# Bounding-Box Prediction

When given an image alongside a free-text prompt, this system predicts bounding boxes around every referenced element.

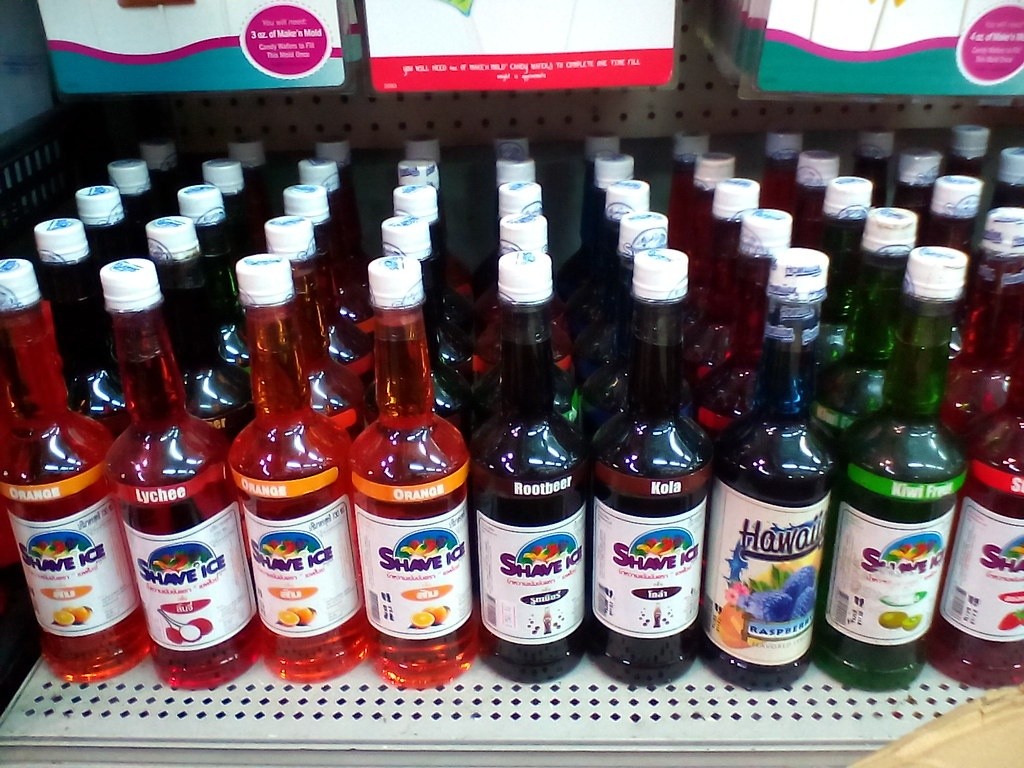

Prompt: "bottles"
[0,124,1024,690]
